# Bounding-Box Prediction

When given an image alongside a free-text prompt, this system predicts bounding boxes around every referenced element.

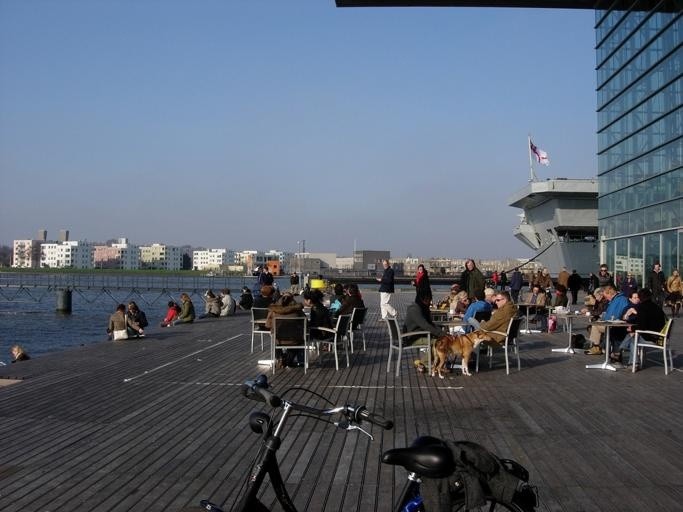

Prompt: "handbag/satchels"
[113,330,129,341]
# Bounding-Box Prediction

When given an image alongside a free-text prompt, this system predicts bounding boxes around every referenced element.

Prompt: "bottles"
[436,300,441,309]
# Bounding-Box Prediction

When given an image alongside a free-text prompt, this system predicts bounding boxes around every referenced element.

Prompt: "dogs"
[431,330,492,379]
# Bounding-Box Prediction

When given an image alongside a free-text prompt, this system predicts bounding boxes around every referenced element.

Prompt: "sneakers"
[583,346,601,355]
[392,312,398,319]
[378,318,385,322]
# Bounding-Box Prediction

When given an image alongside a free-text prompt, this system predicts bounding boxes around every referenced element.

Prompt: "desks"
[431,309,449,336]
[432,320,472,373]
[514,303,636,371]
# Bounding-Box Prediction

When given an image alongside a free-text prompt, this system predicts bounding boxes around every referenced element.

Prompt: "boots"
[672,306,675,317]
[676,304,681,318]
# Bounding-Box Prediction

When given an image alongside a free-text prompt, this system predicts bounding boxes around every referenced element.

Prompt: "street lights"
[296,240,302,288]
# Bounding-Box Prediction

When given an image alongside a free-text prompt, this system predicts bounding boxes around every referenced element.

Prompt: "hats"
[204,290,212,297]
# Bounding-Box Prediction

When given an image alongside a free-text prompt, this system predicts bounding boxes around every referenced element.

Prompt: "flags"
[530,139,548,166]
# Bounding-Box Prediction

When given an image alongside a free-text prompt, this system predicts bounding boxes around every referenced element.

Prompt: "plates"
[595,320,626,324]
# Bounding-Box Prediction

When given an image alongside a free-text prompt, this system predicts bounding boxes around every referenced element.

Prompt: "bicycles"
[200,374,539,512]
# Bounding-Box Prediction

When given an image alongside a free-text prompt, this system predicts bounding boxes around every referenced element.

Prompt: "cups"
[575,310,579,314]
[586,312,591,316]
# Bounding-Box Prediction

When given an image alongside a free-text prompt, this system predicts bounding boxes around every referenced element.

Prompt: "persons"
[450,259,519,349]
[160,293,195,328]
[111,302,148,340]
[253,285,365,367]
[403,259,444,346]
[290,272,299,293]
[510,266,582,316]
[198,286,254,318]
[377,260,399,322]
[10,344,30,363]
[586,262,683,368]
[258,266,274,286]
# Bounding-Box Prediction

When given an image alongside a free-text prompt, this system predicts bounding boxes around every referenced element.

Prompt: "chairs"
[250,307,369,374]
[383,317,433,377]
[474,316,523,375]
[631,317,675,376]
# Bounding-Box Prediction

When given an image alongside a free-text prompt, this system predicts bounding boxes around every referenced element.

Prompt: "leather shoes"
[609,351,622,361]
[536,327,544,332]
[528,319,536,324]
[623,362,639,369]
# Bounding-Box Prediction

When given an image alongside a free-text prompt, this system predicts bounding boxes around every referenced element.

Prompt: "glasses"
[600,270,606,272]
[496,299,504,302]
